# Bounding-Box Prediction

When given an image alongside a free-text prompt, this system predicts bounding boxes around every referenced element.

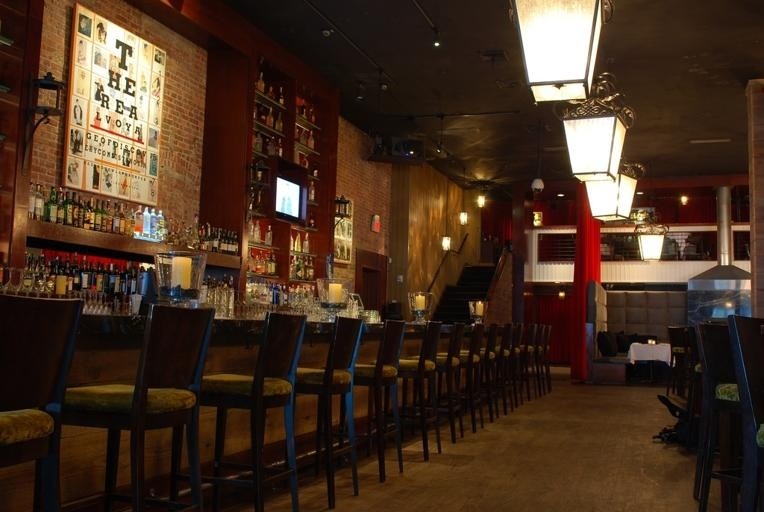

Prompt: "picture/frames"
[61,2,166,207]
[334,194,353,264]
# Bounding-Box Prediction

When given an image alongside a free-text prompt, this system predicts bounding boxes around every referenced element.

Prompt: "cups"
[11,268,24,295]
[24,272,35,297]
[122,296,132,316]
[317,277,352,321]
[408,291,433,325]
[114,296,122,316]
[80,291,112,315]
[35,276,46,298]
[0,268,12,295]
[469,300,488,326]
[132,295,143,314]
[46,278,56,298]
[154,249,206,307]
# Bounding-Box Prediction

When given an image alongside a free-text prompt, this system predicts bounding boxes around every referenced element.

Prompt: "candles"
[475,300,483,315]
[329,283,342,302]
[171,256,192,289]
[415,294,425,309]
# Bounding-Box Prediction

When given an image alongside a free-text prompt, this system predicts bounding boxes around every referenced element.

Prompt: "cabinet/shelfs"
[0,0,45,284]
[28,217,242,269]
[199,53,338,316]
[0,320,504,512]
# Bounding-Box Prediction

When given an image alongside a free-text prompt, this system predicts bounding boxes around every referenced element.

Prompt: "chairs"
[495,323,524,408]
[667,325,691,395]
[402,323,465,443]
[525,323,536,402]
[170,311,307,512]
[693,321,740,512]
[537,323,545,396]
[250,315,365,510]
[544,324,552,394]
[437,324,486,433]
[315,319,409,483]
[367,321,444,462]
[62,303,216,512]
[480,323,514,416]
[455,324,499,423]
[0,295,86,512]
[728,316,764,511]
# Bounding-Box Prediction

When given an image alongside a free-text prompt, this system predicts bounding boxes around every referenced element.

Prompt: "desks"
[627,343,676,368]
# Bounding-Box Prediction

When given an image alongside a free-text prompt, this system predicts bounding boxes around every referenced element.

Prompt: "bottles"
[256,72,265,93]
[48,187,59,223]
[249,186,264,212]
[297,101,317,124]
[124,210,131,234]
[266,107,273,128]
[63,192,73,226]
[277,138,283,158]
[134,205,143,237]
[74,256,82,290]
[291,235,294,251]
[273,284,280,305]
[309,217,315,228]
[113,203,120,233]
[0,253,9,291]
[66,258,73,293]
[28,182,34,219]
[201,272,235,287]
[72,192,78,227]
[280,285,284,304]
[282,285,289,303]
[78,196,83,228]
[254,221,261,243]
[308,181,314,202]
[199,225,239,256]
[119,203,125,235]
[248,248,267,275]
[56,192,64,223]
[290,256,315,280]
[130,208,136,236]
[248,281,272,303]
[249,220,254,241]
[107,200,113,233]
[143,207,150,238]
[303,233,309,252]
[84,200,91,229]
[271,252,278,276]
[156,210,165,239]
[140,266,145,271]
[279,87,284,105]
[251,160,265,181]
[286,285,315,305]
[256,133,276,157]
[275,111,282,131]
[148,267,154,272]
[265,254,272,276]
[265,225,272,247]
[101,200,107,232]
[151,208,157,238]
[25,255,64,275]
[90,198,95,230]
[82,260,137,294]
[294,233,302,251]
[35,184,45,220]
[267,87,274,98]
[308,130,314,150]
[95,199,101,231]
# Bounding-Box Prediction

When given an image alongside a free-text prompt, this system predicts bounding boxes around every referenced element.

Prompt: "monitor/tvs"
[275,176,308,223]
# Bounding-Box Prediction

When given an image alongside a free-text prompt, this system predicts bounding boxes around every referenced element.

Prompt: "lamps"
[584,155,646,222]
[629,211,669,262]
[477,190,486,208]
[440,236,451,253]
[458,212,467,226]
[508,0,614,106]
[553,55,635,182]
[532,178,545,201]
